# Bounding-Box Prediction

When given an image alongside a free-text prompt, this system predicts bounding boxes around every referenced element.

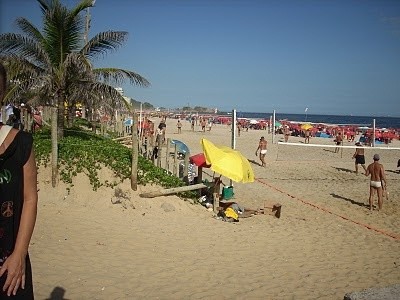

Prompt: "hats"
[373,153,379,159]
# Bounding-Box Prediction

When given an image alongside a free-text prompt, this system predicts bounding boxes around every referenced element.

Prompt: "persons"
[76,100,400,174]
[364,153,388,212]
[0,63,37,300]
[0,100,42,130]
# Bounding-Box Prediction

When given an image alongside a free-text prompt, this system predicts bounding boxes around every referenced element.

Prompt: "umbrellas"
[122,119,134,125]
[200,138,254,185]
[171,138,190,154]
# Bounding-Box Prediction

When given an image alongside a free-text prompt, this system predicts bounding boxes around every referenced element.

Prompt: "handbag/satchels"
[222,185,234,200]
